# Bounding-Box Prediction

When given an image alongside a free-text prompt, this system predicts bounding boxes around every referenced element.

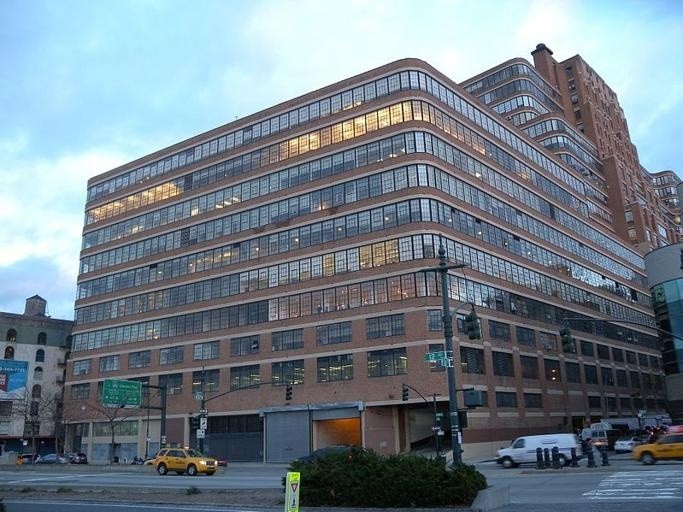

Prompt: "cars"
[142,446,217,476]
[15,452,86,465]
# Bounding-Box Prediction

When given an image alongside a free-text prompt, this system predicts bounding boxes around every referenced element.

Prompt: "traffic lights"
[284,383,293,401]
[466,311,481,340]
[401,386,408,403]
[558,328,573,353]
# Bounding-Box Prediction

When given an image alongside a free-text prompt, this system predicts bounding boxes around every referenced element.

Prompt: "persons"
[585,437,597,467]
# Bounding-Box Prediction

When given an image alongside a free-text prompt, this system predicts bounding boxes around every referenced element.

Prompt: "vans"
[581,413,683,463]
[495,432,584,470]
